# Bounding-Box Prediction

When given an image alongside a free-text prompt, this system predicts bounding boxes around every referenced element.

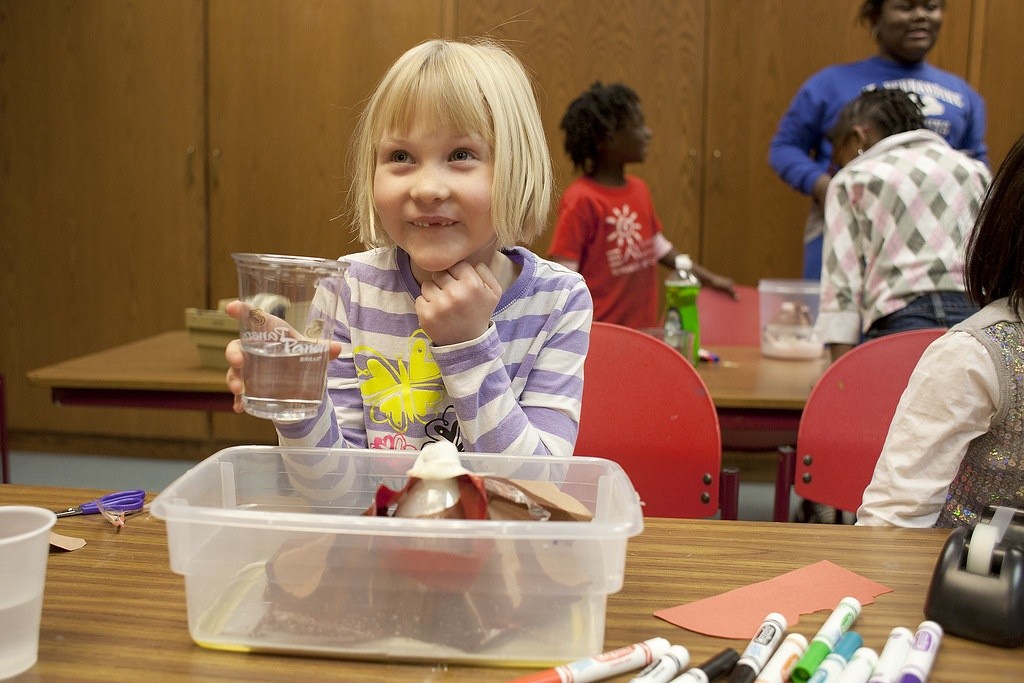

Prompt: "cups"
[758,279,825,360]
[232,253,350,421]
[0,506,59,680]
[645,328,695,365]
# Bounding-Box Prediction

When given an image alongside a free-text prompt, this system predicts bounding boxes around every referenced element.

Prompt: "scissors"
[53,489,145,517]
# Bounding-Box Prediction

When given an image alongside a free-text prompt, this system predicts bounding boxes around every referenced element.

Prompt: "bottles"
[665,254,699,368]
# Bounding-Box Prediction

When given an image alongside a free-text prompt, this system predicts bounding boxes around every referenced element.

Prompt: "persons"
[549,82,741,341]
[226,35,594,515]
[770,1,986,524]
[854,135,1024,529]
[820,89,994,366]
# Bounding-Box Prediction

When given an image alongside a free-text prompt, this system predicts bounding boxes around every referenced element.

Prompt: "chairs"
[793,325,950,515]
[698,286,808,348]
[573,322,723,520]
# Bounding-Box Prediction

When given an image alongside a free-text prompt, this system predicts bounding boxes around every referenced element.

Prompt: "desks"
[26,325,839,444]
[0,483,1024,683]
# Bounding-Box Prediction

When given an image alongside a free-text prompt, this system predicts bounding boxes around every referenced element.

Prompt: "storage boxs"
[148,443,646,665]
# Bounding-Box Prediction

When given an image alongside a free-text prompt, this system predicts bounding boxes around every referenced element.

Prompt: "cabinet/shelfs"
[0,0,444,457]
[442,0,977,317]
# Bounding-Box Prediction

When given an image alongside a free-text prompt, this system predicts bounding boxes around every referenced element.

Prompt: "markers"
[506,595,944,683]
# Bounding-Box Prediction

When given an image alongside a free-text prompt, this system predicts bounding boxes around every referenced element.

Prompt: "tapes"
[966,507,1017,576]
